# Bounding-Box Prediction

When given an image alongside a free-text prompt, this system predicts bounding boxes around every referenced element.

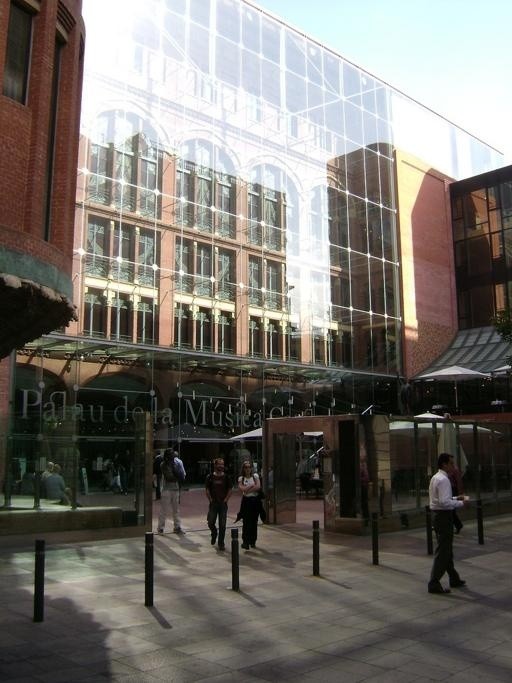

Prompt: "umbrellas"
[427,414,469,478]
[419,365,490,410]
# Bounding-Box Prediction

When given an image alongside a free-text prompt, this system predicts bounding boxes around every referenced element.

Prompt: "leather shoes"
[428,581,466,592]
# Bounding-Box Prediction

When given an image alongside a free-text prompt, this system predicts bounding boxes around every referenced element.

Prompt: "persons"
[103,449,133,495]
[152,449,187,536]
[427,452,466,594]
[205,457,234,551]
[234,461,266,550]
[19,461,73,506]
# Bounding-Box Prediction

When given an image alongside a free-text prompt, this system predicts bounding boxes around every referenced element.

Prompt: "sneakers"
[157,528,185,534]
[211,534,256,549]
